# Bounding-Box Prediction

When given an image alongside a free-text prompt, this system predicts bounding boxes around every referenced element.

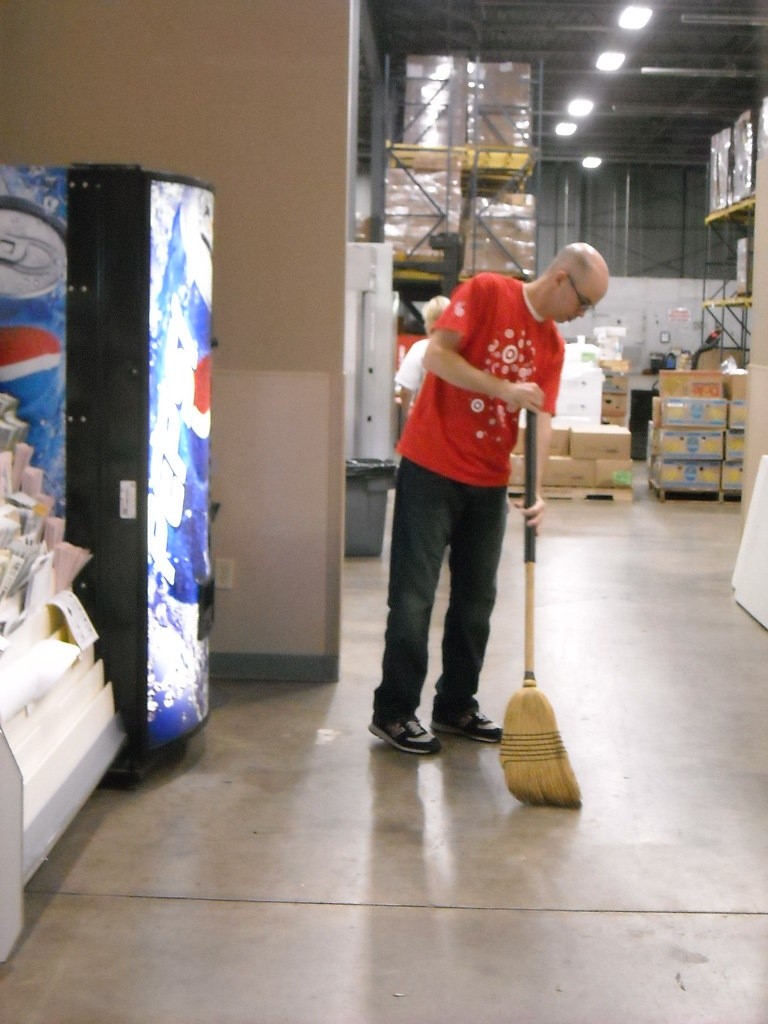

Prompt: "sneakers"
[369,713,442,755]
[430,700,503,743]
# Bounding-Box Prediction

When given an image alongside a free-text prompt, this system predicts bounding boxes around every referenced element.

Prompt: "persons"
[368,241,610,757]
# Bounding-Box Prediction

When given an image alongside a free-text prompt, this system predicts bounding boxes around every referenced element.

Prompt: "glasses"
[566,272,591,313]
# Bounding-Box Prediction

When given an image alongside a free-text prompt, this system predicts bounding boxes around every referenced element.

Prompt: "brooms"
[496,397,584,811]
